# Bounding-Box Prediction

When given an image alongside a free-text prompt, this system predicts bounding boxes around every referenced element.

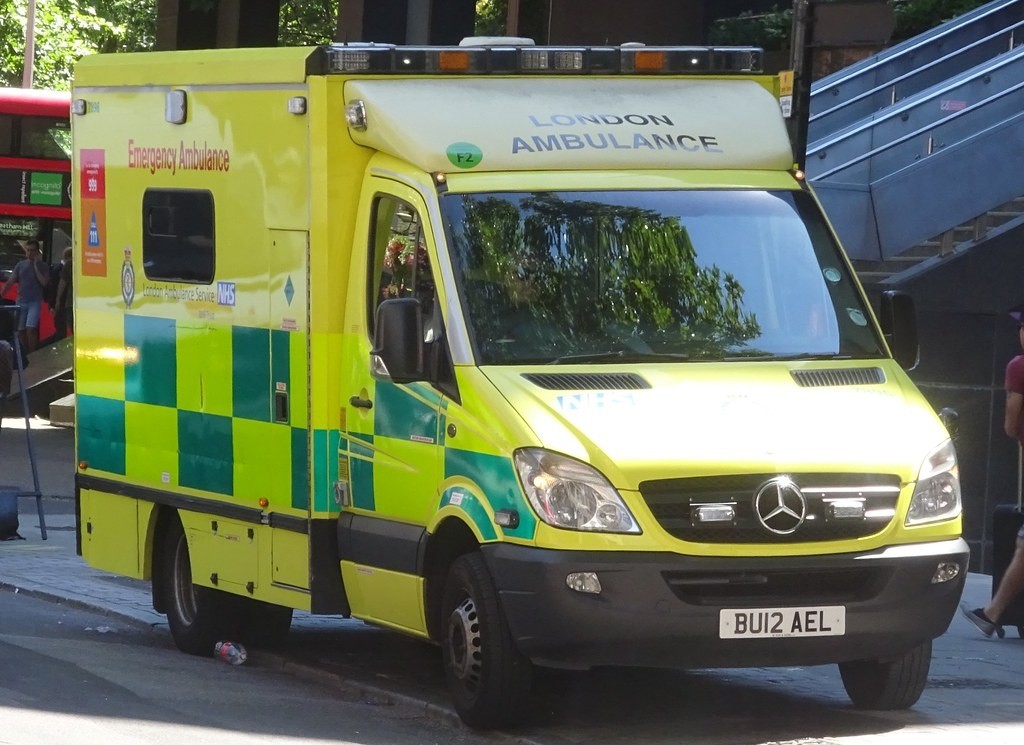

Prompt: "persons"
[503,244,552,311]
[49,248,73,337]
[958,313,1024,637]
[0,239,50,348]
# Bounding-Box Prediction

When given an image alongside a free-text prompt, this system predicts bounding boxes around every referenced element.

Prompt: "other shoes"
[957,598,996,638]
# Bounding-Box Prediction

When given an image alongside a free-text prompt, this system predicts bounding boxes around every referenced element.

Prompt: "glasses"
[1015,323,1024,333]
[508,267,545,283]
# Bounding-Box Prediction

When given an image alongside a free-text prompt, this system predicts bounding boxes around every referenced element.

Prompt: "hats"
[1009,303,1024,323]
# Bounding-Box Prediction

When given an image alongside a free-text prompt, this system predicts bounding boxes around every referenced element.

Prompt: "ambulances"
[70,36,971,734]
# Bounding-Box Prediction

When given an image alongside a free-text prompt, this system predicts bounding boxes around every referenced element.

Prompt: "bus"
[0,87,71,354]
